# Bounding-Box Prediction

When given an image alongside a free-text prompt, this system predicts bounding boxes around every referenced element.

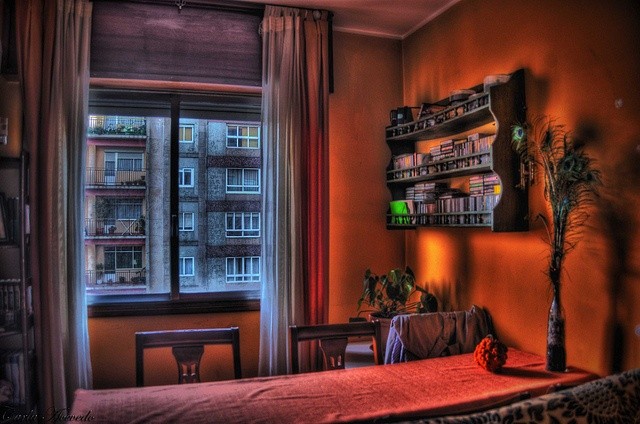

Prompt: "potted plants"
[96,263,104,284]
[91,195,113,236]
[120,174,146,188]
[135,215,145,235]
[355,264,439,361]
[507,110,606,373]
[108,225,116,234]
[136,268,146,284]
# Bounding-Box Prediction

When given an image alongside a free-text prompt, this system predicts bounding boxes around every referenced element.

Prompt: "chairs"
[133,326,242,387]
[285,318,384,376]
[384,304,494,365]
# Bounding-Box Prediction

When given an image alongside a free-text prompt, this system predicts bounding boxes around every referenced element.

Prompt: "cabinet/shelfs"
[0,152,42,423]
[385,67,530,232]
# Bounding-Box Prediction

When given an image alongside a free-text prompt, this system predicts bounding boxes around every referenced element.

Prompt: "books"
[389,173,502,224]
[389,134,497,178]
